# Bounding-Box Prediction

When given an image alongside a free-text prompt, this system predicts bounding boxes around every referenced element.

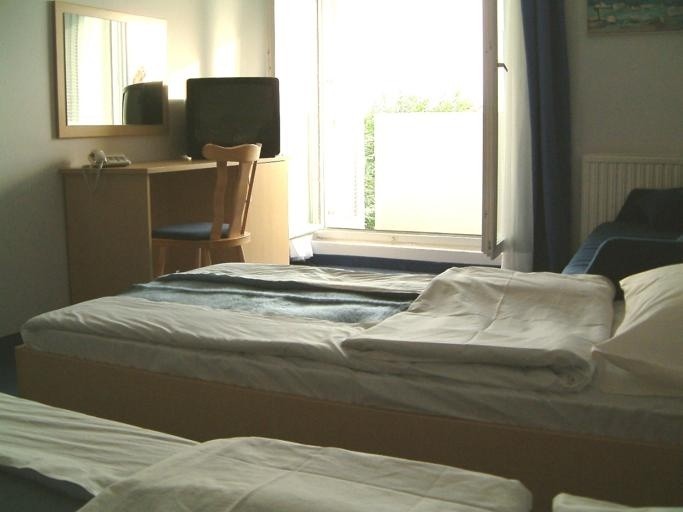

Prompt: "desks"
[59,153,290,308]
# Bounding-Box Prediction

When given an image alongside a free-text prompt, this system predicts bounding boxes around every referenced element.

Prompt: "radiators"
[573,151,683,250]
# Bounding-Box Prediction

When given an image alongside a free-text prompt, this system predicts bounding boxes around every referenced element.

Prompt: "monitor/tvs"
[122,80,162,123]
[185,77,280,157]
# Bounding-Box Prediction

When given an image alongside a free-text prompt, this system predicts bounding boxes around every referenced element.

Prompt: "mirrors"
[52,0,168,139]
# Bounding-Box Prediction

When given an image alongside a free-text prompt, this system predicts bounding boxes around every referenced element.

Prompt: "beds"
[11,257,683,511]
[0,385,680,511]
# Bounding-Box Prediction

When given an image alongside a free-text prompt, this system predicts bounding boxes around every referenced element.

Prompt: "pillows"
[588,261,683,389]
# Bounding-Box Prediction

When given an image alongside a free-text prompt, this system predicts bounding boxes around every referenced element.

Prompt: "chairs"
[147,139,262,281]
[562,185,683,297]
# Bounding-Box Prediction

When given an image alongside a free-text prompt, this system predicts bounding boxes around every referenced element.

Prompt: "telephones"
[88,149,131,168]
